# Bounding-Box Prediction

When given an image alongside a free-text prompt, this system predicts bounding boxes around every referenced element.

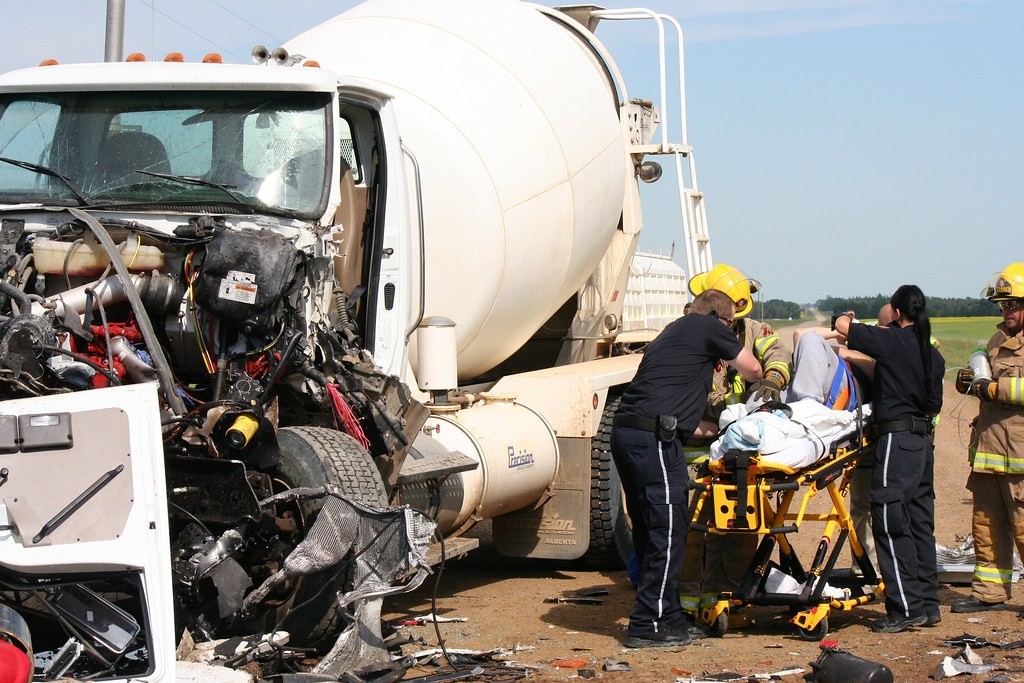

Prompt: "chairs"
[96,126,360,324]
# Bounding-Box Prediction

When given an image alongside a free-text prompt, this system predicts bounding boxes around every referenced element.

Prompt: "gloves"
[955,366,975,395]
[972,378,998,403]
[841,309,855,321]
[745,368,786,404]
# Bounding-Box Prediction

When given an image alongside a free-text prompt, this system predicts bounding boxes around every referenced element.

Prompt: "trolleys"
[672,430,897,640]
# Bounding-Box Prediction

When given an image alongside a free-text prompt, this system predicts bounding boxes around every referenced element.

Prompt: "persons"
[681,263,792,622]
[951,264,1024,618]
[610,290,763,648]
[834,285,945,632]
[711,304,938,580]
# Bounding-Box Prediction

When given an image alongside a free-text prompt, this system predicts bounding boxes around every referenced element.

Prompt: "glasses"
[995,300,1024,310]
[718,316,734,329]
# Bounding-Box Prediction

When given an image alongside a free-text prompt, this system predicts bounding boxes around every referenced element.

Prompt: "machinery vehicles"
[0,0,724,652]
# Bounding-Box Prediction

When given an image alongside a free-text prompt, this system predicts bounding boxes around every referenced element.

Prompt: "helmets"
[689,263,755,320]
[987,263,1024,303]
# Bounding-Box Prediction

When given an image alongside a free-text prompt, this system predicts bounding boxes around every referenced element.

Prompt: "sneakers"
[870,614,930,633]
[677,618,715,639]
[624,624,694,648]
[951,594,1007,613]
[918,609,942,627]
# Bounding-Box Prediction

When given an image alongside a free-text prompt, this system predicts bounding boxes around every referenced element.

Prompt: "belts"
[611,414,660,432]
[874,420,911,436]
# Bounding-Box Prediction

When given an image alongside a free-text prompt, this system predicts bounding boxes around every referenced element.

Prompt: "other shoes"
[847,564,864,584]
